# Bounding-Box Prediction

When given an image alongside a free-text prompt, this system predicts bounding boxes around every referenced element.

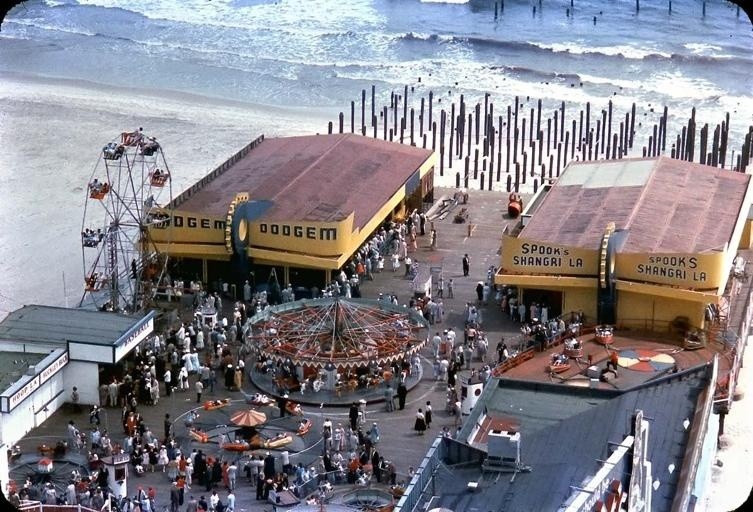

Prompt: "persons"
[82,228,94,247]
[8,386,434,512]
[103,302,110,311]
[334,213,437,292]
[131,259,136,276]
[89,178,107,192]
[153,169,164,180]
[489,264,584,367]
[463,253,470,276]
[100,275,488,429]
[91,274,97,287]
